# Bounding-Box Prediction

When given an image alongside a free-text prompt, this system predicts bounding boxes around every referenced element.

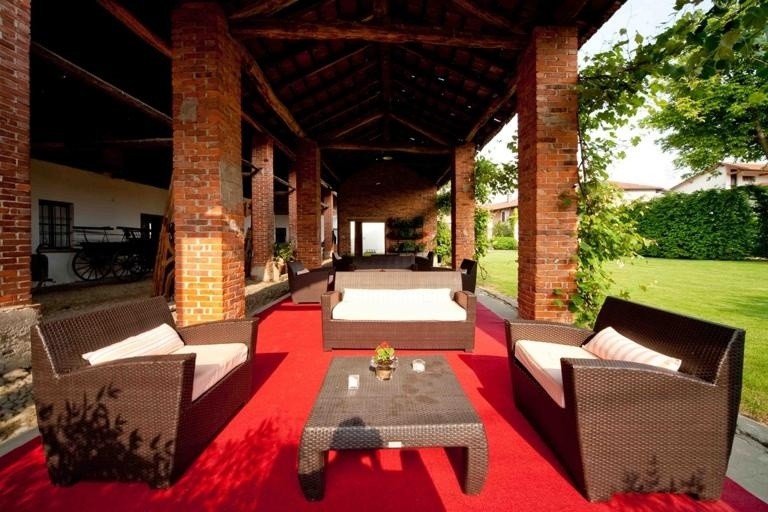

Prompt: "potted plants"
[274,239,297,274]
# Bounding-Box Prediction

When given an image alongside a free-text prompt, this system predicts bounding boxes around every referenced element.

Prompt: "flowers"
[374,341,395,365]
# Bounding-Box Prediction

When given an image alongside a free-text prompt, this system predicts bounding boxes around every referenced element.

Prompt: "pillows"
[80,322,186,366]
[581,326,682,372]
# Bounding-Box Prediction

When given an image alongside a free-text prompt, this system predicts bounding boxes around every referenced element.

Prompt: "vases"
[375,366,396,380]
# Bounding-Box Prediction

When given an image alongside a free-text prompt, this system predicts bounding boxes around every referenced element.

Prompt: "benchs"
[287,251,477,352]
[503,296,746,502]
[30,296,260,488]
